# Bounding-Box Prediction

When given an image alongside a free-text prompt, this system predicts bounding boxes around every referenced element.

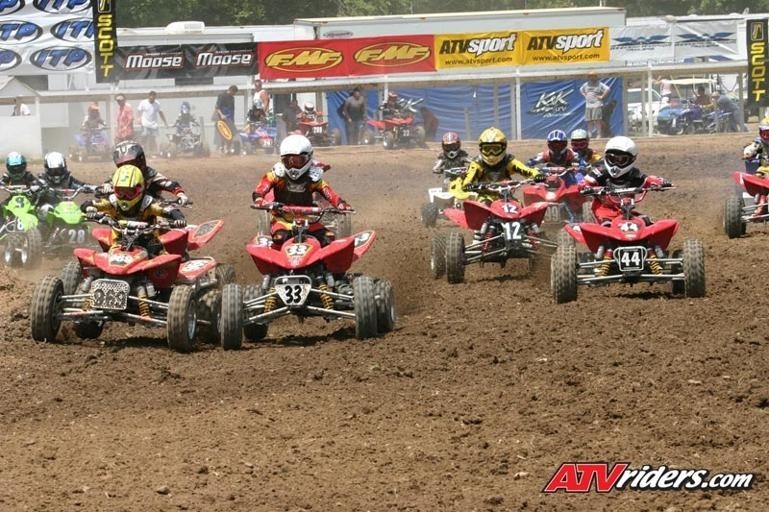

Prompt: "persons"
[12,96,31,117]
[82,78,421,153]
[430,129,674,229]
[250,136,350,247]
[418,105,440,143]
[1,150,41,220]
[97,140,190,206]
[712,90,746,131]
[743,118,769,177]
[654,74,673,105]
[81,167,188,256]
[696,85,710,105]
[33,151,96,206]
[579,71,612,138]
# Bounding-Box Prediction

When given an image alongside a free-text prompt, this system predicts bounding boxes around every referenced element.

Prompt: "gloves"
[534,174,546,183]
[463,183,475,192]
[659,181,672,191]
[102,182,113,195]
[176,192,192,209]
[255,196,269,209]
[173,219,186,228]
[584,186,596,196]
[337,202,352,212]
[86,205,98,220]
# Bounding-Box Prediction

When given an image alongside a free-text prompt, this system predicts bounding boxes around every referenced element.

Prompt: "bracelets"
[601,96,603,99]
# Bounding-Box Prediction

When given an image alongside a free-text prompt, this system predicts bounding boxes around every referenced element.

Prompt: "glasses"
[480,143,506,156]
[7,163,26,173]
[442,142,460,152]
[45,164,67,176]
[760,129,769,140]
[281,151,313,169]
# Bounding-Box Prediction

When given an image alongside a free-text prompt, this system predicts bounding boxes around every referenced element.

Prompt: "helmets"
[304,102,314,114]
[388,90,397,106]
[6,152,27,181]
[758,117,769,146]
[111,140,145,213]
[442,132,460,159]
[44,151,68,184]
[180,104,190,117]
[478,126,508,167]
[280,133,314,181]
[88,104,99,118]
[548,129,639,178]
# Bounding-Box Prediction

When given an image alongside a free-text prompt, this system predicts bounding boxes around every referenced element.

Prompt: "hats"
[585,71,600,79]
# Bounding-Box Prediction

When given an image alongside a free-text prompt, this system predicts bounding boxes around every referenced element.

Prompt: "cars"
[624,85,663,126]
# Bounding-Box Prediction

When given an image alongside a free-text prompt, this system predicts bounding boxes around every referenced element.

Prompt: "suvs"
[212,198,397,351]
[724,157,769,239]
[420,169,705,303]
[656,93,736,135]
[67,97,428,165]
[0,171,220,353]
